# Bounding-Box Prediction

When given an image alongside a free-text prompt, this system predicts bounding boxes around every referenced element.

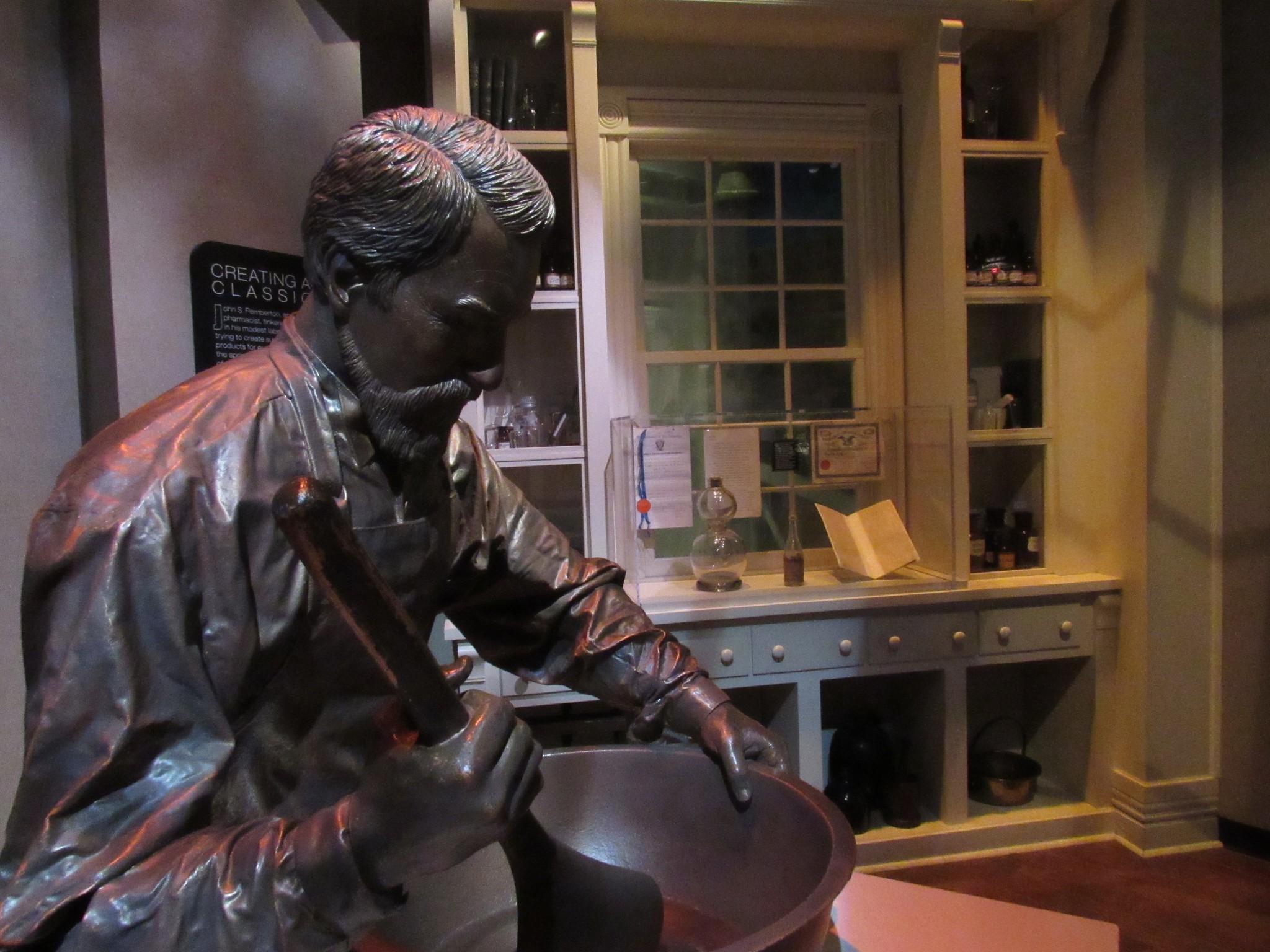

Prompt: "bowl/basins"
[343,741,854,952]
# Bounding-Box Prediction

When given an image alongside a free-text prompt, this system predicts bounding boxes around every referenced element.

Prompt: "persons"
[0,101,790,951]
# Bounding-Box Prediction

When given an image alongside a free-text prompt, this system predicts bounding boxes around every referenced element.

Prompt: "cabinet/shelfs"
[900,13,1062,581]
[408,569,1126,841]
[424,0,612,577]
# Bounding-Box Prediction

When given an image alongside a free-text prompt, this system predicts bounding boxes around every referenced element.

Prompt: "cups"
[885,772,921,826]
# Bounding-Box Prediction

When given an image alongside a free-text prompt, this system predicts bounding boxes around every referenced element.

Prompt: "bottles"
[781,516,805,587]
[970,507,1037,571]
[484,392,578,448]
[537,262,572,288]
[512,84,538,130]
[824,713,890,826]
[968,378,1032,431]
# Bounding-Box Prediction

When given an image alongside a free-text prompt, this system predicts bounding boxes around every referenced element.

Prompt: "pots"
[971,750,1042,804]
